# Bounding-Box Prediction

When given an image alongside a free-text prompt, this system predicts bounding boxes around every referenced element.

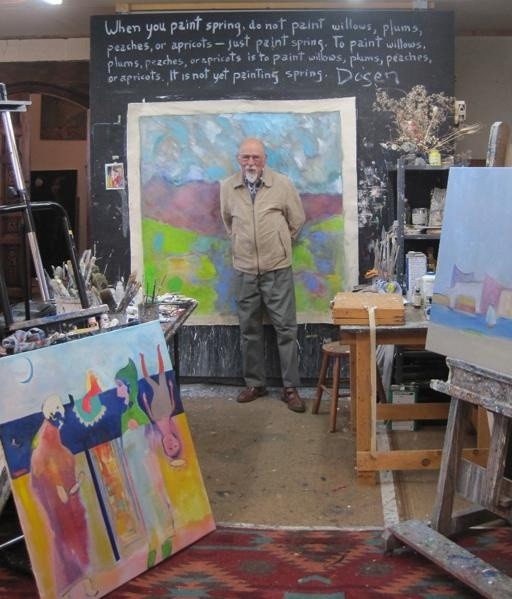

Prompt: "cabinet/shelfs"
[385,155,469,425]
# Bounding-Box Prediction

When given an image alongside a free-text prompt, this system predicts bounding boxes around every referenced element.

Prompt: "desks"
[340,295,493,485]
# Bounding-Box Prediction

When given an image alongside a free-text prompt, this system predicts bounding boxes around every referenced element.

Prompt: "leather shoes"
[281,386,305,413]
[236,385,267,403]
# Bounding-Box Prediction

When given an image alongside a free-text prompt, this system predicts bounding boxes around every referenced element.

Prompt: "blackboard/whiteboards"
[89,8,455,378]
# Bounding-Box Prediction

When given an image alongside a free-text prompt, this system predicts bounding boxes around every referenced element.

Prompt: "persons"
[219,137,308,413]
[116,356,178,568]
[139,345,182,460]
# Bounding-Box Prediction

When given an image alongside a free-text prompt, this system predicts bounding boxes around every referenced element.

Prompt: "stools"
[312,340,386,434]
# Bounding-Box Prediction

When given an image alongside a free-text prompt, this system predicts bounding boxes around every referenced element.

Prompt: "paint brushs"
[374,232,400,282]
[44,243,167,314]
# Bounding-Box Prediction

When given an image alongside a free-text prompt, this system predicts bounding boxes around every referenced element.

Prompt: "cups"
[412,208,428,225]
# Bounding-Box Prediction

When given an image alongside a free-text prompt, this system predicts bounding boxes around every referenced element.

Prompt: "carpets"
[0,522,512,599]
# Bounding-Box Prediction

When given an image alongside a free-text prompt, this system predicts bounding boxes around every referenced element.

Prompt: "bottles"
[403,198,411,225]
[107,281,124,304]
[428,149,442,166]
[87,310,109,331]
[411,289,422,308]
